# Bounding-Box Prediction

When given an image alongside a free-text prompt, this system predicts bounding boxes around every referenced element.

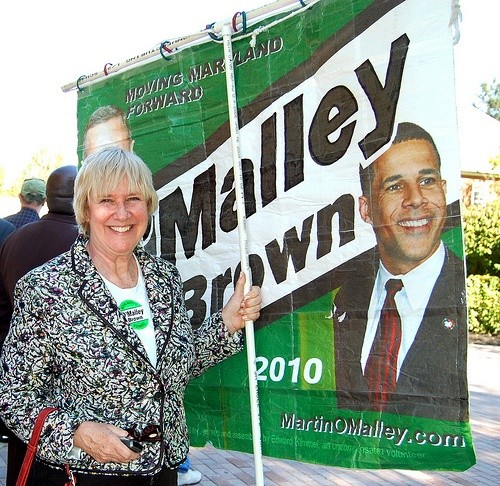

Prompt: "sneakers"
[177,470,201,486]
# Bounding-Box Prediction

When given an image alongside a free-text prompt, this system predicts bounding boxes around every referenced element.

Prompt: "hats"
[20,178,47,200]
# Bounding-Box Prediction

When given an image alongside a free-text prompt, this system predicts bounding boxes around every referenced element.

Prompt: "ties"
[363,278,404,416]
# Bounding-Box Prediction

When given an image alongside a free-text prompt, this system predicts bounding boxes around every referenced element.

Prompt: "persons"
[0,165,79,356]
[3,177,47,229]
[82,106,134,167]
[177,455,201,485]
[0,148,262,486]
[333,121,469,422]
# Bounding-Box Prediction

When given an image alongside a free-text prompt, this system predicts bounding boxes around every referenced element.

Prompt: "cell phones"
[120,439,143,453]
